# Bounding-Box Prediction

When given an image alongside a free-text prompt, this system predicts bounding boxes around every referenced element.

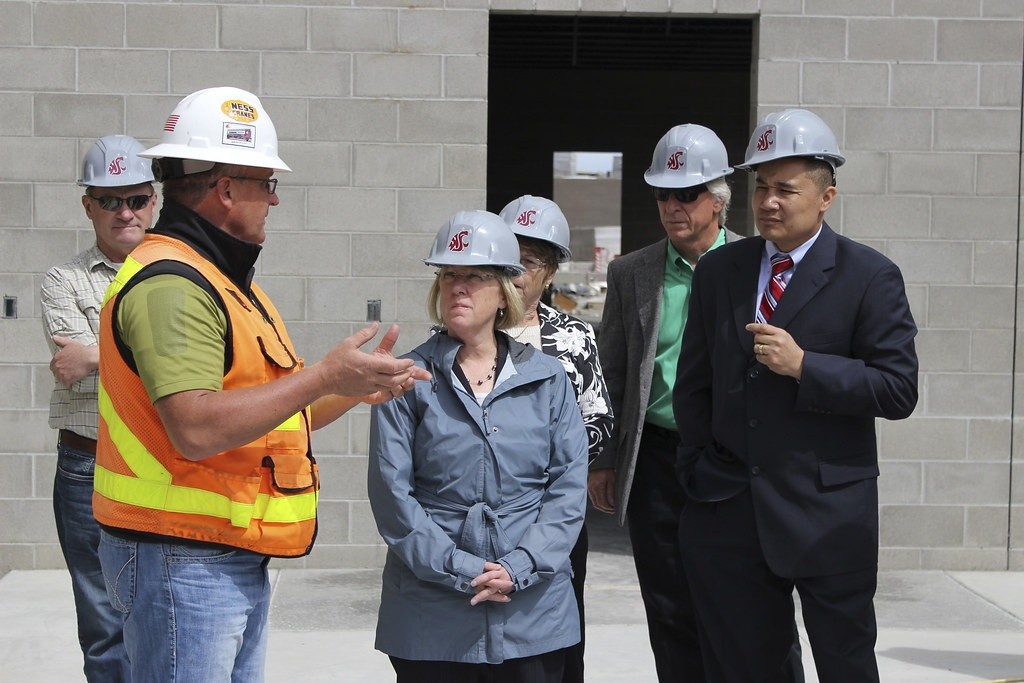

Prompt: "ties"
[755,253,794,325]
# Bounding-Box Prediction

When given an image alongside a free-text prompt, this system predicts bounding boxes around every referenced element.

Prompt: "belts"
[60,429,97,455]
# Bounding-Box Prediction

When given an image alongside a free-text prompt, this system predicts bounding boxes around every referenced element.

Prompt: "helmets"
[643,124,734,189]
[137,87,293,183]
[77,135,155,187]
[734,108,846,170]
[499,195,572,263]
[421,210,526,277]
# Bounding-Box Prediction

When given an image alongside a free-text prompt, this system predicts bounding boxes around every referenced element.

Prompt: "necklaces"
[465,355,499,386]
[503,324,530,339]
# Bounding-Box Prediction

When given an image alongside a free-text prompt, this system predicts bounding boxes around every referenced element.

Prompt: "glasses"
[434,268,495,287]
[209,175,278,195]
[653,186,708,203]
[520,254,546,270]
[87,193,152,211]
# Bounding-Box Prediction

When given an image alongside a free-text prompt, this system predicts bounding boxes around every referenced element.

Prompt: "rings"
[758,343,764,355]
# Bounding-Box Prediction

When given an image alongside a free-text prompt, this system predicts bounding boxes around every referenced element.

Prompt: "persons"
[91,87,432,683]
[671,108,919,683]
[40,134,159,683]
[368,209,590,683]
[497,195,614,683]
[597,123,749,683]
